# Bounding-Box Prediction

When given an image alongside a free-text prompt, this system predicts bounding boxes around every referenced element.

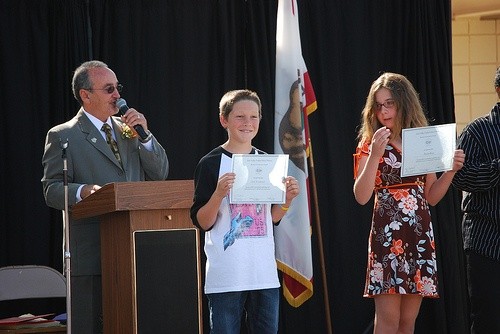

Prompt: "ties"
[99,123,122,165]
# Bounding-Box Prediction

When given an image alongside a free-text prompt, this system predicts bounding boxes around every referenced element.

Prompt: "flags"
[273,0,317,308]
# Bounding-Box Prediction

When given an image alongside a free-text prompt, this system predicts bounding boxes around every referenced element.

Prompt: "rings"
[137,114,140,119]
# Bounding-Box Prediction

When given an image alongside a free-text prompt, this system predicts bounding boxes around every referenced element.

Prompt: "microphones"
[115,98,149,140]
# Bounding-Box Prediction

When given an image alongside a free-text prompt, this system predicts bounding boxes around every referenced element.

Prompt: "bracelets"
[279,204,289,211]
[138,130,152,143]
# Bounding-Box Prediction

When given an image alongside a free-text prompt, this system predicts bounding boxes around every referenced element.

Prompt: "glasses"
[84,84,123,93]
[373,101,395,111]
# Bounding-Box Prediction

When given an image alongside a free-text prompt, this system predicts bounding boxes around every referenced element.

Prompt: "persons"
[450,66,500,334]
[191,89,300,334]
[42,61,169,334]
[353,73,465,334]
